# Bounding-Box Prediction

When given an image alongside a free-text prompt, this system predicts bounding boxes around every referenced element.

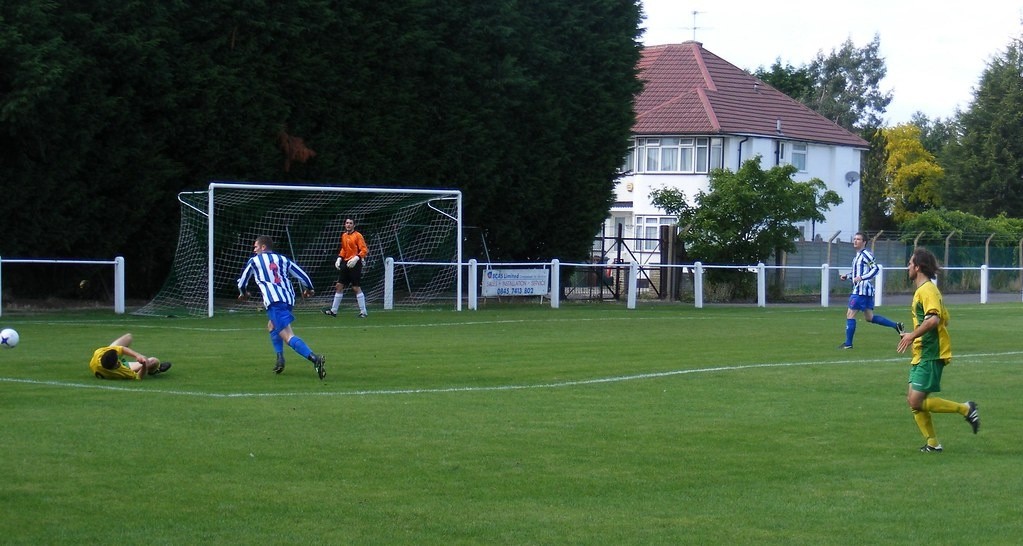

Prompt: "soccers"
[0,328,20,349]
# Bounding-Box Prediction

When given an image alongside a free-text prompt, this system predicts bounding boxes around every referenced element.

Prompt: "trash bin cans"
[570,256,611,287]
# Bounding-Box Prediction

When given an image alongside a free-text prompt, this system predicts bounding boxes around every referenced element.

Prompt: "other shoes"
[148,362,171,375]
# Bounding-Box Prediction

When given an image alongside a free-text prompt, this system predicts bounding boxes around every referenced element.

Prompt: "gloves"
[335,257,342,270]
[346,256,360,268]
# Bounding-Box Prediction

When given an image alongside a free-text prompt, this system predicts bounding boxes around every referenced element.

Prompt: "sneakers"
[895,322,905,339]
[315,355,326,379]
[964,400,978,434]
[835,343,853,349]
[273,363,284,374]
[320,307,336,318]
[359,313,367,319]
[919,444,942,453]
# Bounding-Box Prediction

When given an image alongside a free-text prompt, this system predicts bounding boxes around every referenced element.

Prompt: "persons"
[237,235,327,380]
[896,246,981,455]
[836,232,905,350]
[89,332,171,380]
[322,217,369,318]
